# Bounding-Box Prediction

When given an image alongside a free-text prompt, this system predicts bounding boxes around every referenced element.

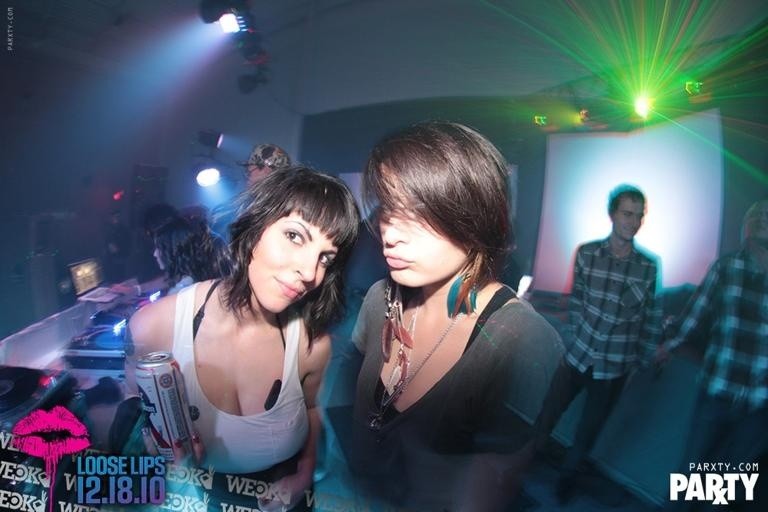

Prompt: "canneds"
[134,351,199,462]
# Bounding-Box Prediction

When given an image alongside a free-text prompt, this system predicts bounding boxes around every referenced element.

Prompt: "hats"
[235,144,291,171]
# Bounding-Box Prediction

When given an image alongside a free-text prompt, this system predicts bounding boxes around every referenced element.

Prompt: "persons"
[103,118,768,512]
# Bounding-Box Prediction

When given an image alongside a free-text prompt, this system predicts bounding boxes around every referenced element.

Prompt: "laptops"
[68,257,124,303]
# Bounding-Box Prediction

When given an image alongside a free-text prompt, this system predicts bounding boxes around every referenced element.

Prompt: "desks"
[1,278,137,414]
